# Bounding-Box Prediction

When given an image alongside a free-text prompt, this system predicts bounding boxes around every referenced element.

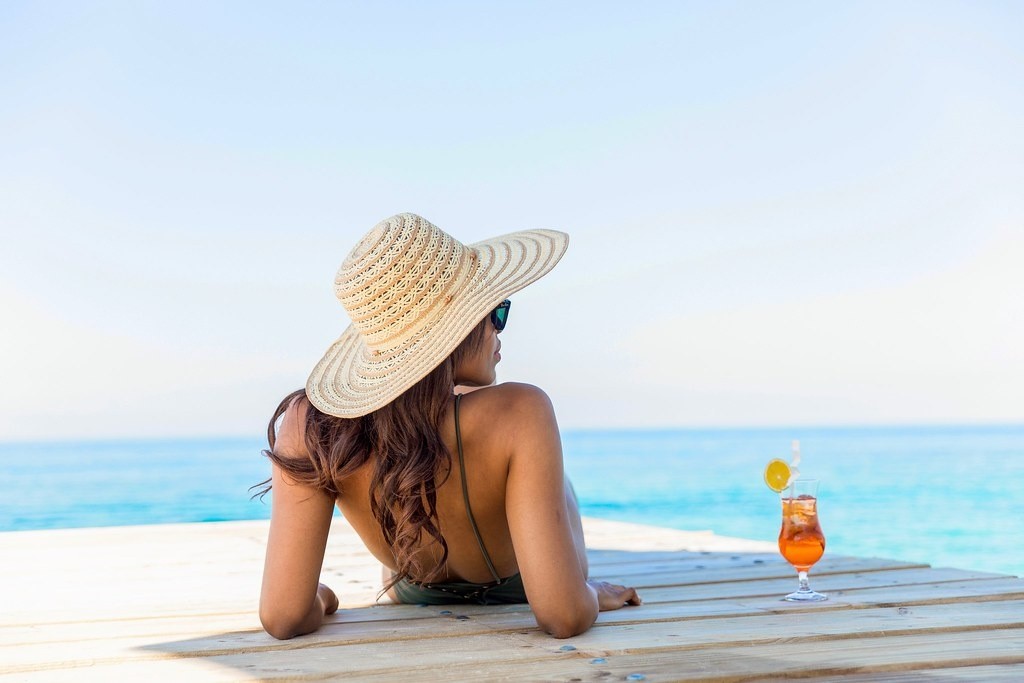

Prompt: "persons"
[247,212,642,640]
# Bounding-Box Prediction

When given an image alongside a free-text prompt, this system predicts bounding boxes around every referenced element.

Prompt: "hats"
[305,213,569,419]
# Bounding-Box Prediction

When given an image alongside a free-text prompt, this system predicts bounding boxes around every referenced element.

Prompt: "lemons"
[764,459,791,492]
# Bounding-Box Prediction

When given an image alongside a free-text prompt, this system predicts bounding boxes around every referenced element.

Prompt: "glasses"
[490,300,512,331]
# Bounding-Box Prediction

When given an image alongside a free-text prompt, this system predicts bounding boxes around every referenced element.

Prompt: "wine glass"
[779,477,829,603]
